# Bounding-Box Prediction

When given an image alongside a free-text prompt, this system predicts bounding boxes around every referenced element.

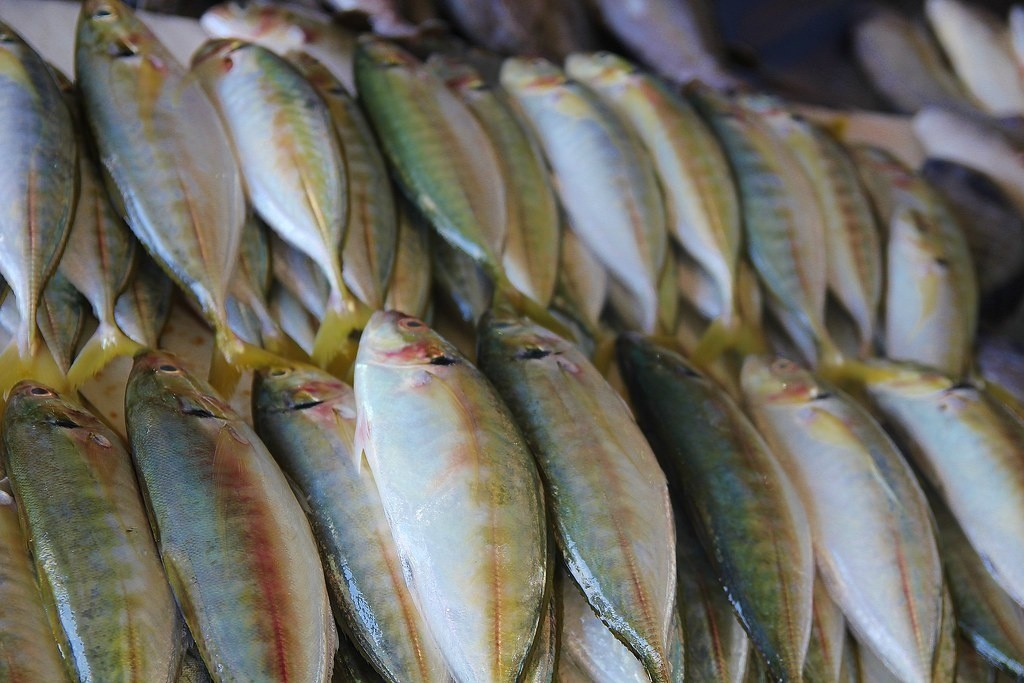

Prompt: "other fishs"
[0,0,1022,683]
